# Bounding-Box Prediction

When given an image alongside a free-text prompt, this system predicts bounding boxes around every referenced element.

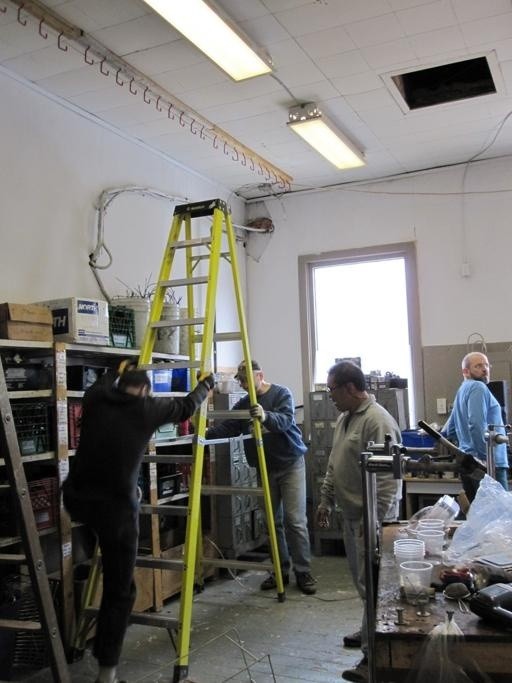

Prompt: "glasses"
[326,385,344,393]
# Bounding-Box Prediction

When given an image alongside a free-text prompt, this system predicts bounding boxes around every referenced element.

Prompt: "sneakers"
[342,659,369,683]
[343,631,361,647]
[261,572,289,590]
[296,573,318,594]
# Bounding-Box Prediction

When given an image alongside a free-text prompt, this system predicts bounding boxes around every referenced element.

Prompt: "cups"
[390,516,446,607]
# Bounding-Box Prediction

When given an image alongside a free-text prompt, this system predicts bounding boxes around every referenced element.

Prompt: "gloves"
[249,403,266,423]
[197,371,217,392]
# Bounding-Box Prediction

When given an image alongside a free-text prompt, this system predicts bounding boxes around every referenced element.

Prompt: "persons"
[319,362,406,681]
[439,350,510,502]
[204,358,313,594]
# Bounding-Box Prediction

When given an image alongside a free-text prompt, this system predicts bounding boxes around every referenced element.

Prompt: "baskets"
[0,572,62,681]
[10,402,50,456]
[137,463,150,499]
[69,402,83,449]
[0,477,60,537]
[109,305,136,348]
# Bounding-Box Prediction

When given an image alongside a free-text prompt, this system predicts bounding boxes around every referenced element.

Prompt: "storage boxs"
[37,298,114,347]
[5,300,56,344]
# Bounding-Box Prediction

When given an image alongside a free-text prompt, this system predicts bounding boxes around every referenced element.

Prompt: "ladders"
[69,199,284,682]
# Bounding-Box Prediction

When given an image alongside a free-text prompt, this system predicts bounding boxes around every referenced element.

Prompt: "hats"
[234,360,261,378]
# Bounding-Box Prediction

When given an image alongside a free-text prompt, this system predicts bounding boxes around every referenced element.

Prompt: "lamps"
[289,101,367,170]
[147,0,273,87]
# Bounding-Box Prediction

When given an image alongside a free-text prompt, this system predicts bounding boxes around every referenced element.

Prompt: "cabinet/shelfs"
[2,341,69,683]
[307,384,404,554]
[203,431,269,573]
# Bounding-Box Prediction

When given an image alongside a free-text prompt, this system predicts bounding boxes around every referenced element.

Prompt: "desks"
[403,476,465,520]
[369,519,512,682]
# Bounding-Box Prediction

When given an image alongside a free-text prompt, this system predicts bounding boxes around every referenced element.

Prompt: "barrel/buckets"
[400,428,449,460]
[110,298,151,350]
[179,306,203,357]
[151,302,180,356]
